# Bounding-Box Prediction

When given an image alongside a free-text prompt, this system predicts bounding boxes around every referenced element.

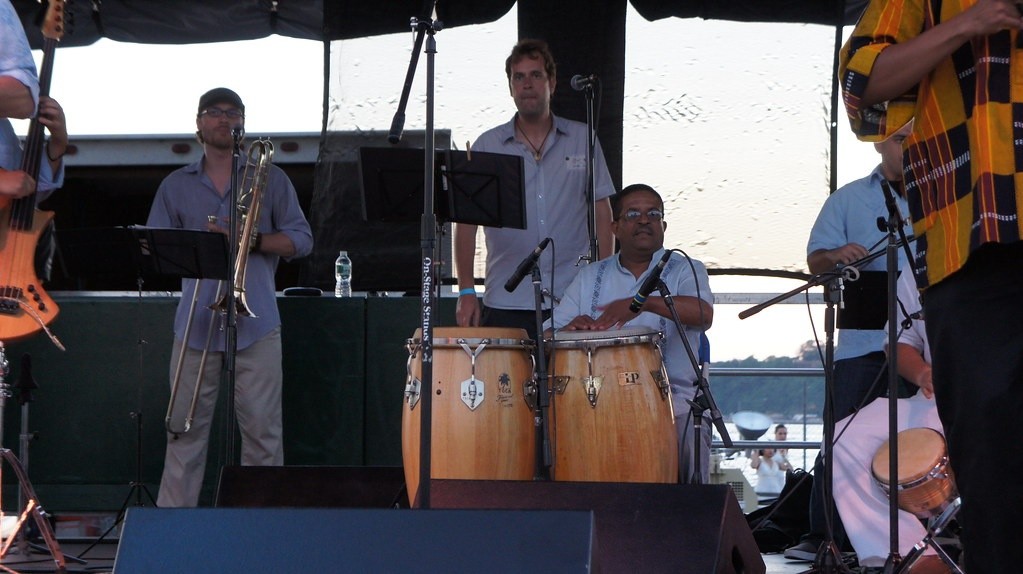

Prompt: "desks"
[3,291,486,515]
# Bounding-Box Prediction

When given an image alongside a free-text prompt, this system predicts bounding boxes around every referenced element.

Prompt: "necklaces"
[516,120,552,160]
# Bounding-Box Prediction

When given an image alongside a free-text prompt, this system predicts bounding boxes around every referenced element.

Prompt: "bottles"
[335,250,351,297]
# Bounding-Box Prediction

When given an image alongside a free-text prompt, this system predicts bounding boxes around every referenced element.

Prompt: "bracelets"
[254,230,263,256]
[45,139,67,162]
[458,287,476,296]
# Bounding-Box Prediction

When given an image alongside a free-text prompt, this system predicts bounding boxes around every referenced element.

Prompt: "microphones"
[910,309,926,319]
[629,250,672,313]
[231,125,243,139]
[503,237,549,293]
[571,74,598,91]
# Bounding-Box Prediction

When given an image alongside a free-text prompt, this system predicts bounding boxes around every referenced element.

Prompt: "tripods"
[73,412,157,557]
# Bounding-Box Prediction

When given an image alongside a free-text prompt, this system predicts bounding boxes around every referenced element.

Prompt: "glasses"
[198,106,245,120]
[612,208,665,223]
[776,432,786,436]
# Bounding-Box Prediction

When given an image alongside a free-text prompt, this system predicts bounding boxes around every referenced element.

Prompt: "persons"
[810,0,1023,574]
[542,184,712,484]
[806,115,956,574]
[146,86,313,508]
[752,440,785,493]
[455,39,619,340]
[0,0,68,552]
[770,423,793,473]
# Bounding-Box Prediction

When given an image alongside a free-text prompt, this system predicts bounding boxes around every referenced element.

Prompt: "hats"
[198,87,243,114]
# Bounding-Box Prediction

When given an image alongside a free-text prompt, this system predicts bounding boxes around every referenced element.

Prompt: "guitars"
[1,1,78,351]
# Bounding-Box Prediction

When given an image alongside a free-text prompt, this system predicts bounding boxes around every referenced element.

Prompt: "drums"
[546,325,680,485]
[400,325,540,509]
[870,426,959,520]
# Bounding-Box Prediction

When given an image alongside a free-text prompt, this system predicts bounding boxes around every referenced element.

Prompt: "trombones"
[162,137,277,435]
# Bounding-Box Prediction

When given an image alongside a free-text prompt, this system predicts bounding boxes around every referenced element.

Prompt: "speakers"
[114,463,766,574]
